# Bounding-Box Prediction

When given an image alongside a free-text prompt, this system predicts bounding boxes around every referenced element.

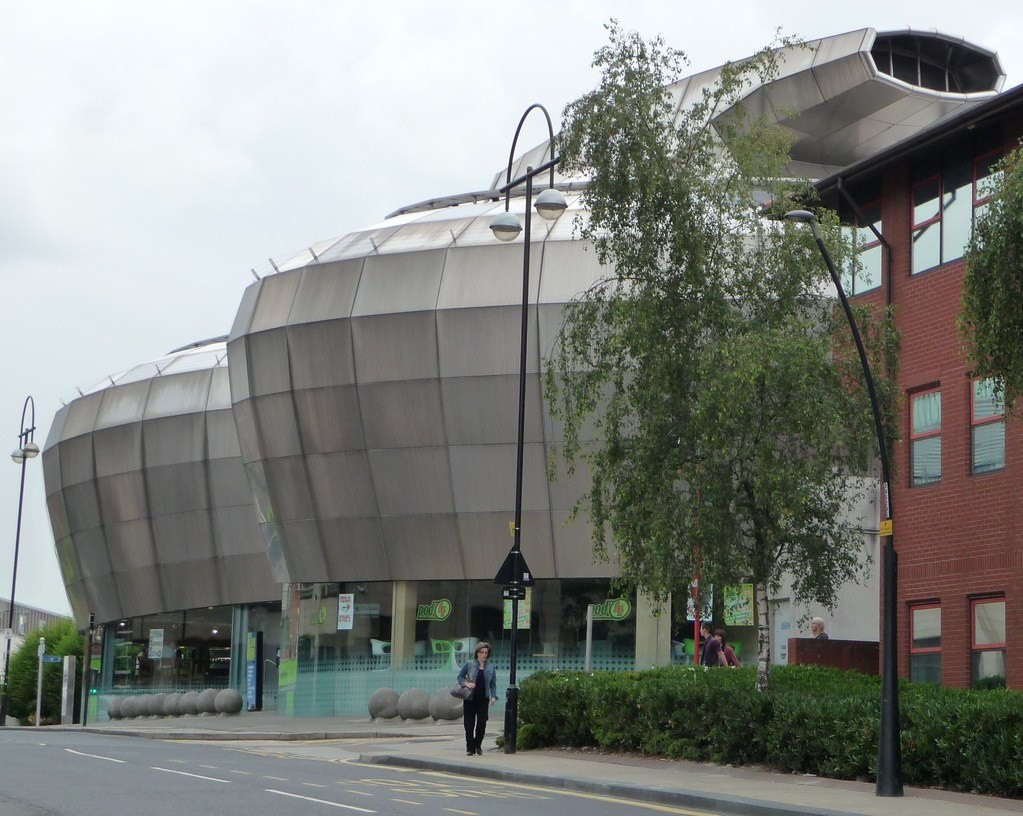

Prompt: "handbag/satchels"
[450,660,474,701]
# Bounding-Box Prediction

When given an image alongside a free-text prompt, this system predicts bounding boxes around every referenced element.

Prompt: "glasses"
[479,651,489,654]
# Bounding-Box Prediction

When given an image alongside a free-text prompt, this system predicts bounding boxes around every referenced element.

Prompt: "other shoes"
[476,745,482,755]
[467,751,473,756]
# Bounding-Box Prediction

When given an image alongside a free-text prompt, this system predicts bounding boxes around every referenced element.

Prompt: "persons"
[457,642,498,755]
[714,630,740,666]
[699,621,727,666]
[810,617,828,639]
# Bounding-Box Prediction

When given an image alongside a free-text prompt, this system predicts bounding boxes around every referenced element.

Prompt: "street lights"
[0,395,41,729]
[789,209,904,798]
[488,103,571,750]
